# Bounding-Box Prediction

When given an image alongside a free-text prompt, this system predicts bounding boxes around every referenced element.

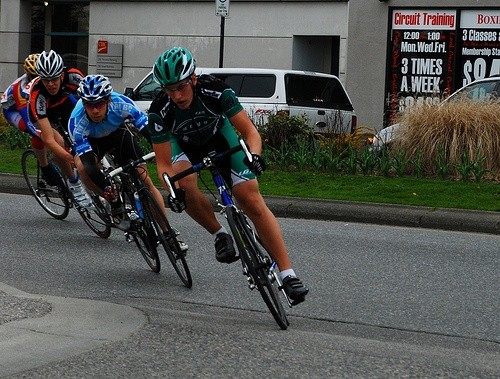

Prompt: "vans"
[123,67,356,142]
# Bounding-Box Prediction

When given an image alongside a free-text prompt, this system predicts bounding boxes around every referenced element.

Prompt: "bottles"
[134,191,144,219]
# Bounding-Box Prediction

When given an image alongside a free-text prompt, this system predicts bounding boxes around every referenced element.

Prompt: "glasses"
[42,77,60,83]
[83,100,109,109]
[161,78,190,92]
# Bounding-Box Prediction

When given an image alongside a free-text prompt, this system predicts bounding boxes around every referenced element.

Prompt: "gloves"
[243,154,267,177]
[168,187,187,213]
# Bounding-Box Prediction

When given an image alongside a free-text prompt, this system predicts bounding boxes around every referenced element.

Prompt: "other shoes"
[39,162,60,186]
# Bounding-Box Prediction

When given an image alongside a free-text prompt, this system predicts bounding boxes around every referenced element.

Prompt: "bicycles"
[52,117,126,238]
[162,134,305,330]
[102,151,193,288]
[21,149,73,219]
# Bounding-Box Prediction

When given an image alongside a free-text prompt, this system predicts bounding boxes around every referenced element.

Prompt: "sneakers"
[165,233,188,253]
[214,232,236,262]
[67,178,92,207]
[110,200,131,231]
[283,274,309,299]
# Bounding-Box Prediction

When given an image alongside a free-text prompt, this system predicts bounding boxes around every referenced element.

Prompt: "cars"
[366,77,500,152]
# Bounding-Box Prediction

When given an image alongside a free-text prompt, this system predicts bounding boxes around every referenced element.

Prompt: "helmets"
[77,74,113,104]
[152,46,196,87]
[35,50,65,80]
[23,54,43,76]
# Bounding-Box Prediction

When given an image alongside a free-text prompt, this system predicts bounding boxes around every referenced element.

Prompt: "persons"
[148,47,309,299]
[31,50,92,206]
[68,75,189,253]
[0,53,63,186]
